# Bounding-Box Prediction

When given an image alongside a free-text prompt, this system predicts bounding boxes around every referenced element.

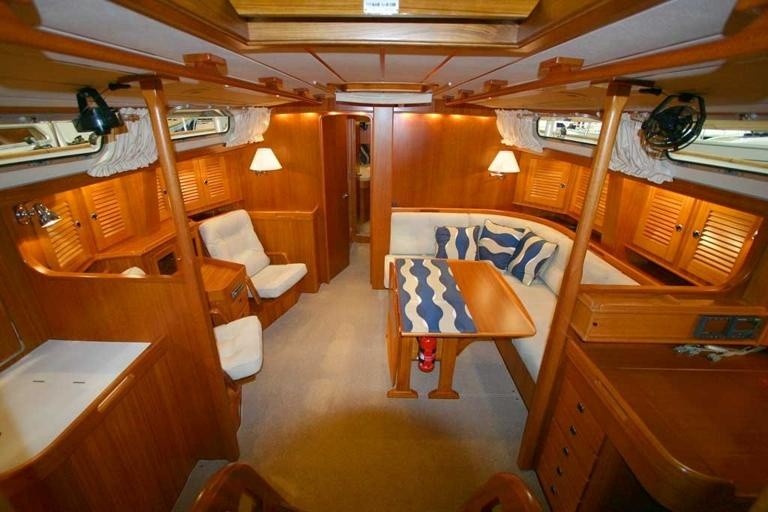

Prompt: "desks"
[386,257,537,399]
[534,339,768,511]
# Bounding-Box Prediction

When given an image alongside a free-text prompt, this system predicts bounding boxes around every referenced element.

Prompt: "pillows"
[506,225,558,286]
[478,218,523,272]
[435,226,480,261]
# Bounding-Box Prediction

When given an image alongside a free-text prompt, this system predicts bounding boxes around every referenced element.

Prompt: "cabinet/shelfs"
[198,256,250,326]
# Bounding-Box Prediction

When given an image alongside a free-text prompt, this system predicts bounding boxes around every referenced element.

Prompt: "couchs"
[389,205,667,413]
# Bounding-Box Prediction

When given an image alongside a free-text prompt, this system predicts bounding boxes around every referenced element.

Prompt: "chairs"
[101,267,264,383]
[195,209,308,330]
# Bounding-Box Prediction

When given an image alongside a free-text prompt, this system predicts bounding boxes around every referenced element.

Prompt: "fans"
[638,93,707,158]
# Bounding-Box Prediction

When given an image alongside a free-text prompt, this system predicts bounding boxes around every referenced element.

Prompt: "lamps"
[249,147,282,176]
[15,203,62,228]
[487,150,521,178]
